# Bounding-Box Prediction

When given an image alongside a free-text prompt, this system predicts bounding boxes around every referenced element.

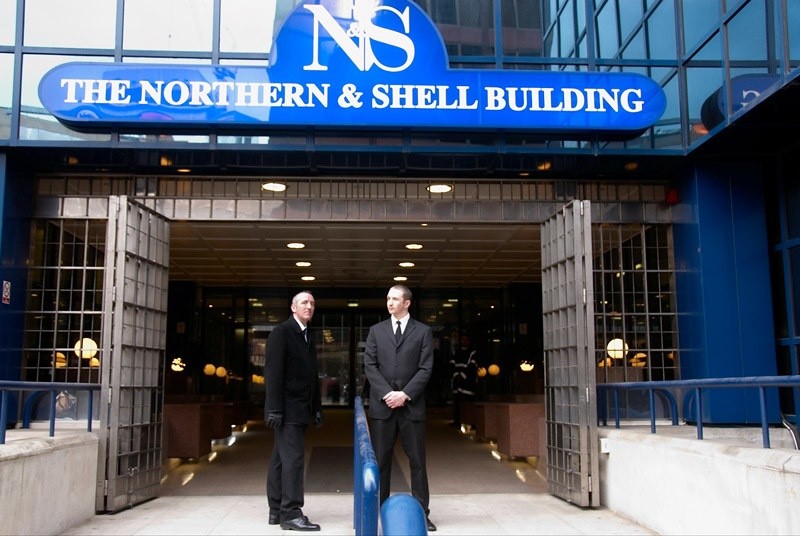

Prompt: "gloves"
[266,412,282,431]
[314,411,323,428]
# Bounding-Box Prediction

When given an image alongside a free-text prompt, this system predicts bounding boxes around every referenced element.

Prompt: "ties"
[394,320,402,344]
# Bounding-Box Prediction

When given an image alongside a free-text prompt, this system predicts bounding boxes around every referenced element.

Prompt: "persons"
[263,291,320,531]
[364,285,437,532]
[450,334,478,429]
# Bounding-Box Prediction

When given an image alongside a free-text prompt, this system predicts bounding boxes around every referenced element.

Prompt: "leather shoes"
[268,513,309,524]
[280,518,321,531]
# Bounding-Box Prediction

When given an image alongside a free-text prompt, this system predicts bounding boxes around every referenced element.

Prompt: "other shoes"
[426,519,437,532]
[449,421,460,426]
